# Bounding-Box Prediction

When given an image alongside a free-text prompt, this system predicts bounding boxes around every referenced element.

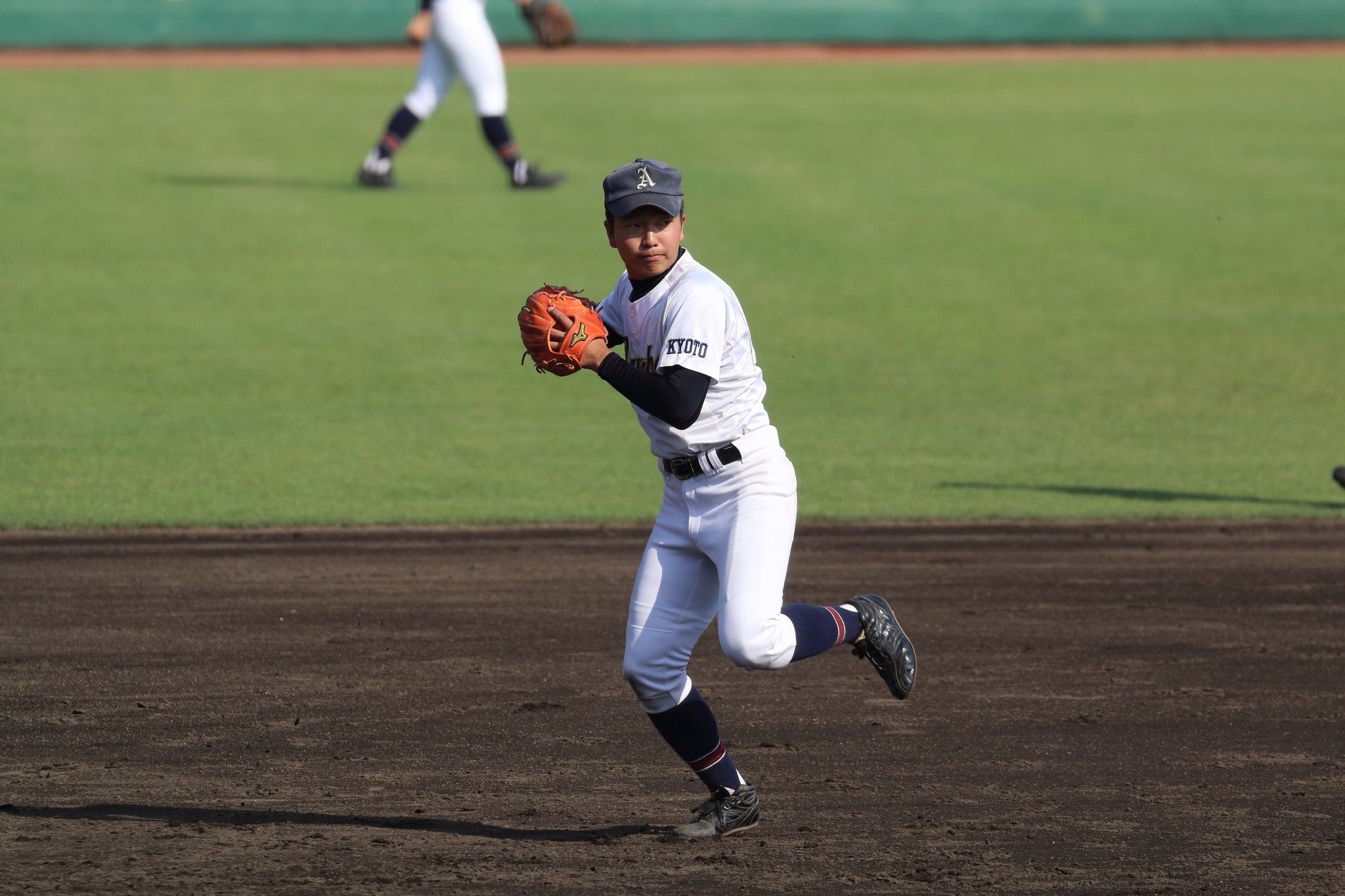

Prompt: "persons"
[516,157,919,842]
[355,0,569,194]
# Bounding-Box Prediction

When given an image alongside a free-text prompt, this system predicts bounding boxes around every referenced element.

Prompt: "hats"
[603,159,684,220]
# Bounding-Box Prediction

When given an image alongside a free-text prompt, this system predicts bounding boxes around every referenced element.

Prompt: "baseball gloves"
[517,282,607,378]
[520,0,576,50]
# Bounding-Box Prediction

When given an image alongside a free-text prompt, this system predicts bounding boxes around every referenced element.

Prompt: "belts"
[662,445,742,479]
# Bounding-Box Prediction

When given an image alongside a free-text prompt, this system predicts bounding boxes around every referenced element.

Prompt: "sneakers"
[845,594,918,700]
[511,167,564,189]
[355,167,398,190]
[673,782,759,839]
